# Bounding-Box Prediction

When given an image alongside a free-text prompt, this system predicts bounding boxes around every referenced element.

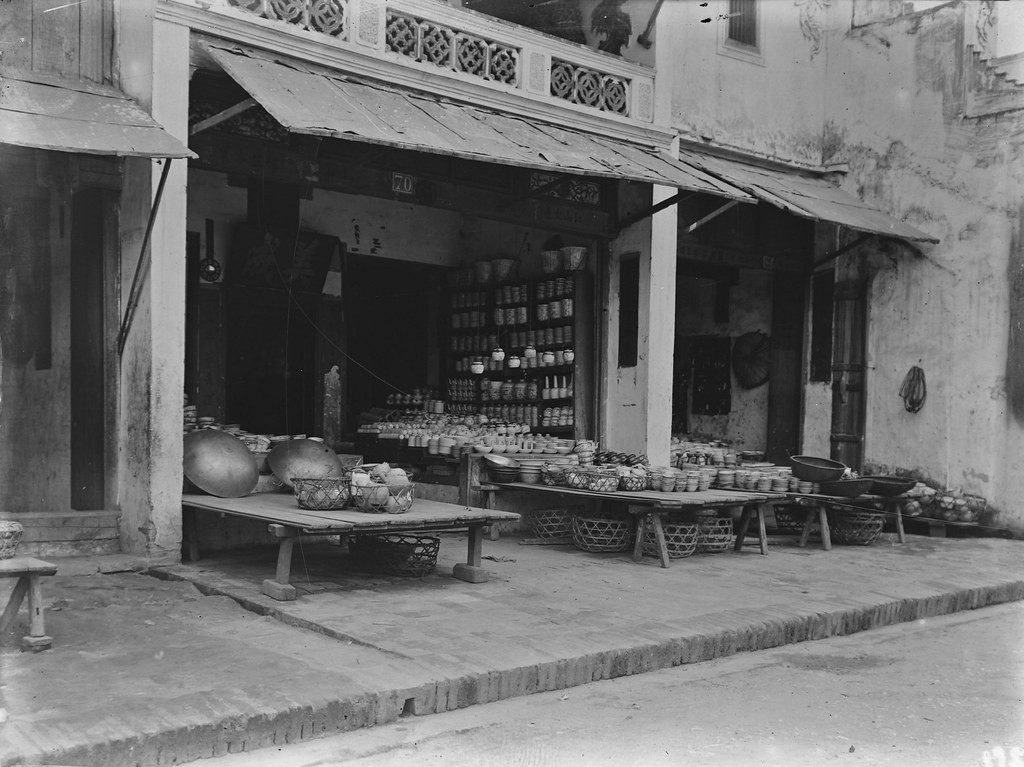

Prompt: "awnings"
[190,40,760,236]
[0,63,199,354]
[678,150,942,274]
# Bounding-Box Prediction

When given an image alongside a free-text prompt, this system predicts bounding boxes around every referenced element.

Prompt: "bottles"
[480,403,538,427]
[480,377,537,400]
[428,399,443,413]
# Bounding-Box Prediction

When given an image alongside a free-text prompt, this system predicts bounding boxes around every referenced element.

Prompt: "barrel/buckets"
[472,260,492,287]
[493,259,520,281]
[561,246,589,271]
[540,250,562,273]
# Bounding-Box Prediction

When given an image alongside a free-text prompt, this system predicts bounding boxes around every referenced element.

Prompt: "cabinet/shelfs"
[444,270,596,441]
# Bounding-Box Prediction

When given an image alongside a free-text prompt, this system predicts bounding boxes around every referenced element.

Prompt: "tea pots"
[351,468,370,495]
[447,377,476,412]
[573,438,599,465]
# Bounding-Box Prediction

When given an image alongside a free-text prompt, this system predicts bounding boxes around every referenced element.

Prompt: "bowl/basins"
[671,434,766,468]
[184,393,324,453]
[482,454,547,483]
[494,275,573,325]
[474,445,571,454]
[650,469,821,494]
[407,435,473,461]
[903,496,985,522]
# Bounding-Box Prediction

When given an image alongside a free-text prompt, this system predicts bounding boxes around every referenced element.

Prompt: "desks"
[0,551,58,637]
[182,494,522,584]
[481,480,787,565]
[711,486,942,551]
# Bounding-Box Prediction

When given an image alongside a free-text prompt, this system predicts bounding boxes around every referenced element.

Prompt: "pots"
[184,430,260,498]
[790,455,919,497]
[266,440,344,488]
